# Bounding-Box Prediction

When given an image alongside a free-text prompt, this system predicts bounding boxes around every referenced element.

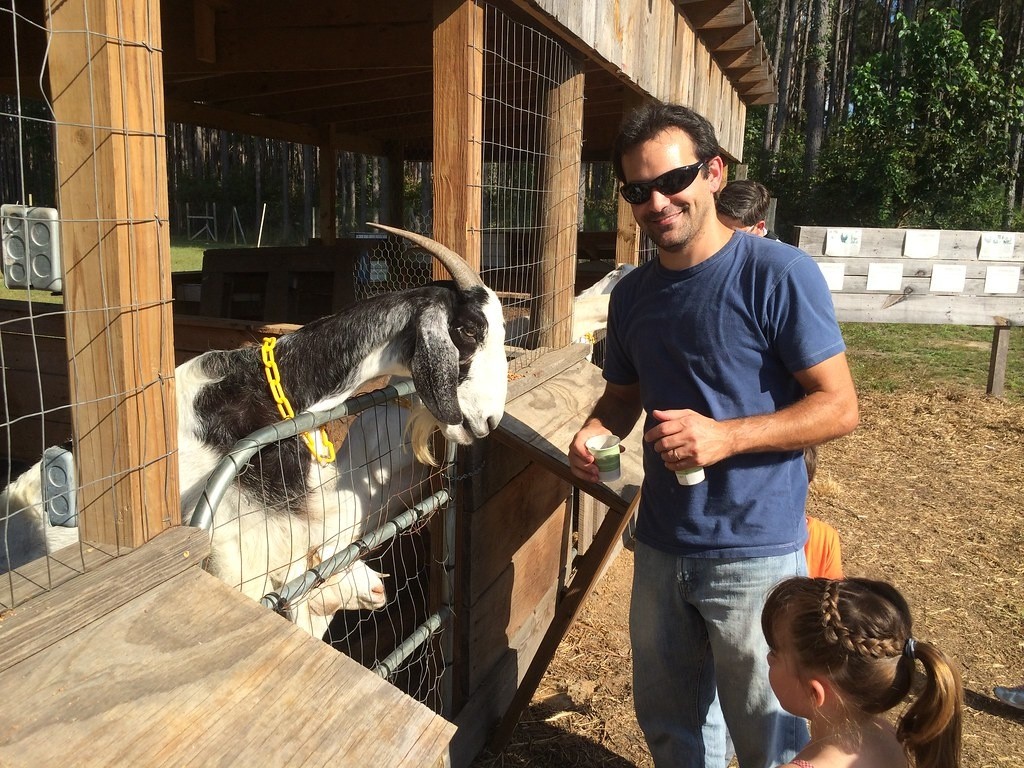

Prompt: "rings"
[673,448,681,461]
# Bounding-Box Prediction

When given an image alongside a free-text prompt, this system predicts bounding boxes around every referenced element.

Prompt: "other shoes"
[993,685,1024,709]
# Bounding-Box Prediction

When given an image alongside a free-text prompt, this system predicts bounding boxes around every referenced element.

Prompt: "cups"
[586,435,621,482]
[675,467,705,485]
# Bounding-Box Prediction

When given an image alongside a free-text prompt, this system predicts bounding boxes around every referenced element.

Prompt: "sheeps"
[2,222,508,640]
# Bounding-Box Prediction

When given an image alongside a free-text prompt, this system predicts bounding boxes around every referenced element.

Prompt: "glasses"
[619,157,710,206]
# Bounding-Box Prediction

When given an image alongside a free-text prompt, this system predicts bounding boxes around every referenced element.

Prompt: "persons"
[715,180,781,242]
[762,575,963,768]
[568,99,860,768]
